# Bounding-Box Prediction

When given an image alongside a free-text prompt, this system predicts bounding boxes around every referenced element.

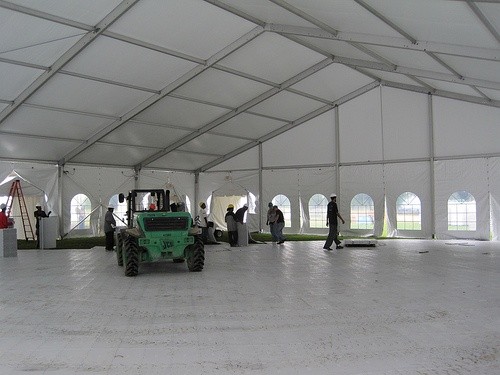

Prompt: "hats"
[244,204,248,207]
[107,204,114,209]
[0,203,6,209]
[200,202,203,207]
[228,204,234,209]
[330,193,336,197]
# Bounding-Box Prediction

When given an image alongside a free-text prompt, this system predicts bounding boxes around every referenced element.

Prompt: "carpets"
[16,231,399,250]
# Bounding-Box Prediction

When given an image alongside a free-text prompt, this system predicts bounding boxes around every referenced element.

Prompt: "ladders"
[6,180,35,241]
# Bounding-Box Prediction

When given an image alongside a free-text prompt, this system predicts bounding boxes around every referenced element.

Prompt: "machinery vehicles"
[115,189,205,277]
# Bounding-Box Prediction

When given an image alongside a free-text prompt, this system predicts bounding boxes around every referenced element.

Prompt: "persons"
[225,204,239,247]
[266,202,285,244]
[0,203,15,229]
[34,206,51,249]
[198,202,209,245]
[323,193,345,251]
[235,204,248,224]
[104,203,116,251]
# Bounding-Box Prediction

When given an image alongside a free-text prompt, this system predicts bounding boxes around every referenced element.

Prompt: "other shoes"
[336,245,344,249]
[323,247,332,250]
[277,241,284,244]
